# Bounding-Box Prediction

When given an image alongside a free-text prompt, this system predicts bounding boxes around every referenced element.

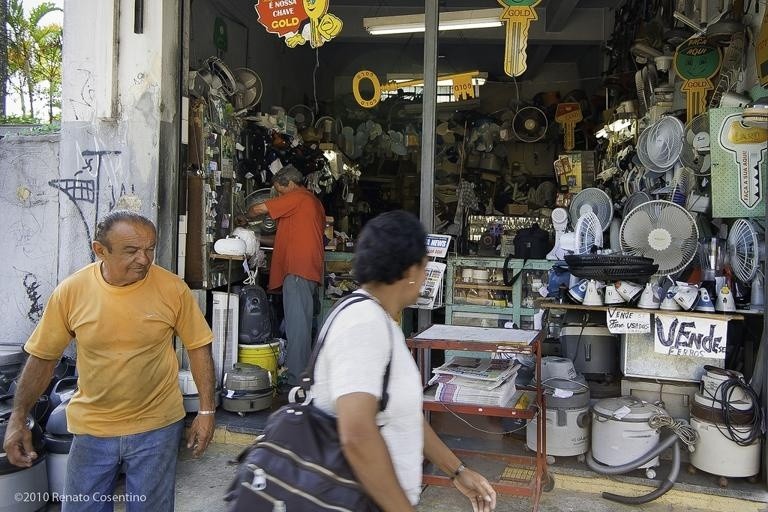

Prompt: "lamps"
[387,72,489,86]
[362,1,506,37]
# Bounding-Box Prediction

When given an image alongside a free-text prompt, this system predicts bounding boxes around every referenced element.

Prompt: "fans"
[242,188,279,235]
[513,107,548,144]
[568,114,768,306]
[204,55,263,112]
[289,105,339,143]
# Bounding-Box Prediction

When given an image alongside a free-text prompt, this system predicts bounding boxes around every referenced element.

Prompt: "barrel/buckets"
[239,341,280,400]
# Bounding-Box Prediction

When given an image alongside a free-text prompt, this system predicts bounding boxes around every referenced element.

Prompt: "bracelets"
[449,461,468,481]
[198,410,218,416]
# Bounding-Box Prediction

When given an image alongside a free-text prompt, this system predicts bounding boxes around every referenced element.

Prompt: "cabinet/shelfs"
[405,324,553,512]
[317,252,557,363]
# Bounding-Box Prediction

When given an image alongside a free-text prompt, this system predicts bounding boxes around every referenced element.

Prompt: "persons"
[3,208,216,511]
[237,164,325,397]
[313,209,496,512]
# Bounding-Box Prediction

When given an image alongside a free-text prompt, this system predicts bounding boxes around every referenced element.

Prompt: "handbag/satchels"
[222,292,394,512]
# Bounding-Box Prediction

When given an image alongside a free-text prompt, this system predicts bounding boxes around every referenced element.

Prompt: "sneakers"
[276,383,299,394]
